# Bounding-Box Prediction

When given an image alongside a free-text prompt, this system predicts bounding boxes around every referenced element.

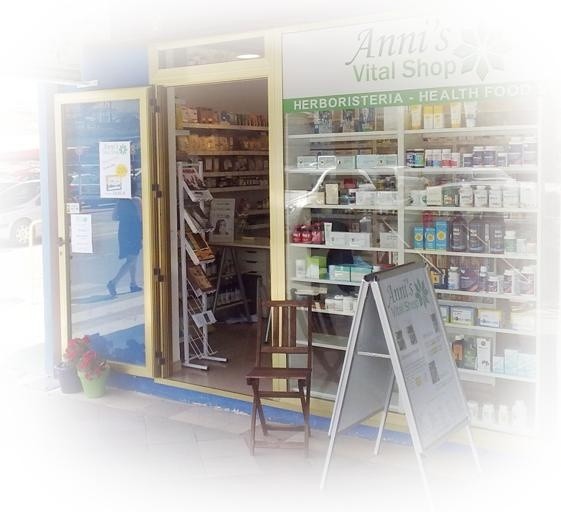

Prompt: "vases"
[60,333,110,382]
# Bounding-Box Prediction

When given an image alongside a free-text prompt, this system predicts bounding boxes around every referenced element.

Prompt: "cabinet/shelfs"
[289,220,329,245]
[450,332,466,371]
[202,253,243,312]
[461,338,478,371]
[256,197,269,208]
[237,176,260,187]
[234,111,266,127]
[403,135,538,296]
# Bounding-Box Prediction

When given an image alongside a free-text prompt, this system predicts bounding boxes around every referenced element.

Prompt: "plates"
[175,122,269,321]
[284,104,543,438]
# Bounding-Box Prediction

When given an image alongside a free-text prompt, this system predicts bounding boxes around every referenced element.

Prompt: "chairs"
[129,285,143,292]
[106,281,117,296]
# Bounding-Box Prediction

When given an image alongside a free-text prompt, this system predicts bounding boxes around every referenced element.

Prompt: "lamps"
[243,299,313,458]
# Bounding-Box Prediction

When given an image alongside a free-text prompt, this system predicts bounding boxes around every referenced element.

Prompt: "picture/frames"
[207,236,270,324]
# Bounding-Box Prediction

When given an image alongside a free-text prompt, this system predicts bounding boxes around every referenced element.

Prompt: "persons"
[214,219,230,235]
[107,180,144,297]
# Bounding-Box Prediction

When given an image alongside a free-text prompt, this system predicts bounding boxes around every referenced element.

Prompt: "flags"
[54,362,110,397]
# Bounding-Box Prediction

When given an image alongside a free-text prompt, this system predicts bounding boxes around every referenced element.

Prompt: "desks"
[66,144,93,199]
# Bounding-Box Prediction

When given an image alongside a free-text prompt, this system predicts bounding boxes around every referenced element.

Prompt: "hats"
[4,161,141,248]
[284,160,519,231]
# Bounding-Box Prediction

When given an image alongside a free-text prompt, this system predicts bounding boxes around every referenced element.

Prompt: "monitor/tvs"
[181,164,221,328]
